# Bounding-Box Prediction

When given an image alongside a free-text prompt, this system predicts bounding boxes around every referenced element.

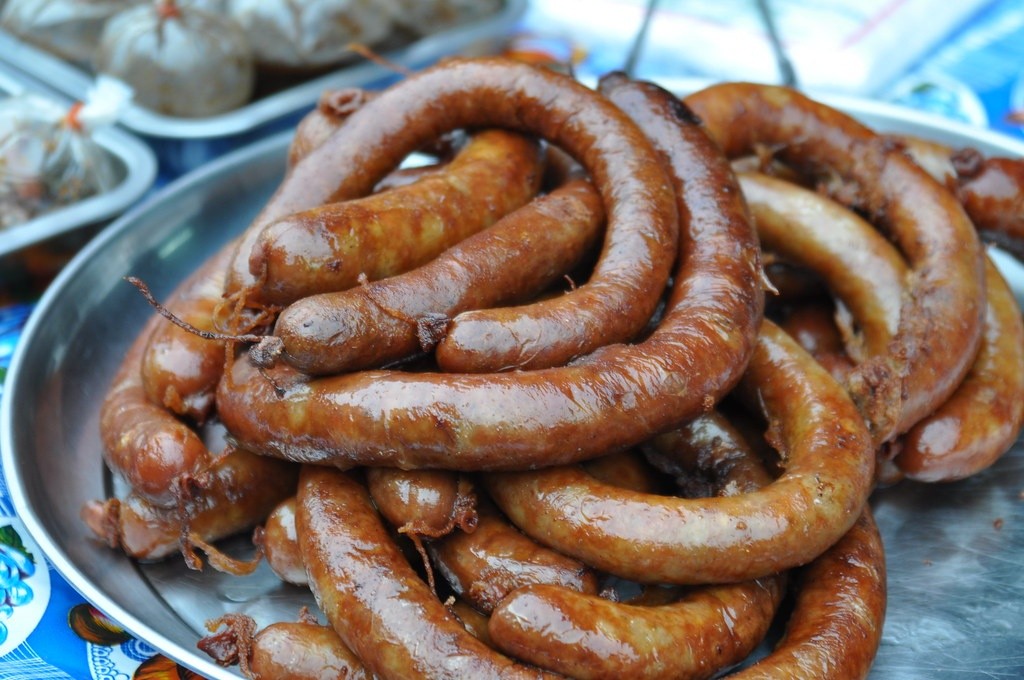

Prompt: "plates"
[0,0,533,177]
[1,75,1024,680]
[0,55,156,258]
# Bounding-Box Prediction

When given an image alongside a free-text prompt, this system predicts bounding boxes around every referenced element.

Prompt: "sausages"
[81,48,1024,680]
